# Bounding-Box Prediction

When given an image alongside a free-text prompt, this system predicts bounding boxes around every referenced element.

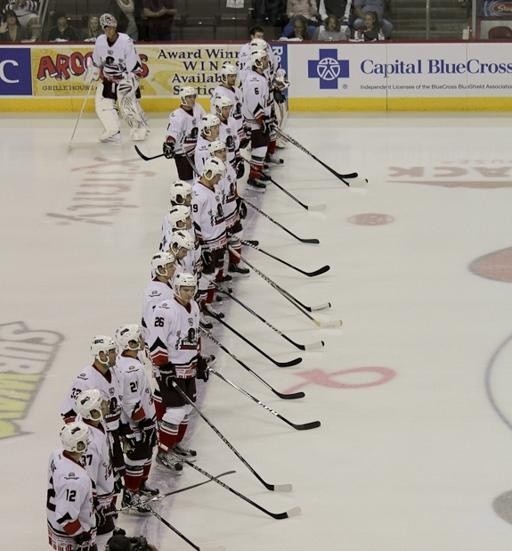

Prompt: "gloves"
[236,197,247,219]
[195,353,209,383]
[163,136,176,159]
[159,361,177,388]
[138,418,157,448]
[95,506,115,527]
[235,157,245,179]
[256,112,279,129]
[73,527,98,551]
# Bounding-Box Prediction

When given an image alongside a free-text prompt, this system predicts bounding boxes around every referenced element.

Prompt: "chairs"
[37,0,280,43]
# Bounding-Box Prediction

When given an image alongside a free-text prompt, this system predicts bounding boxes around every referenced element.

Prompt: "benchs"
[385,0,466,38]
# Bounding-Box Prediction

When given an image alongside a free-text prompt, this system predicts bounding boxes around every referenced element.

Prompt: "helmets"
[250,50,267,65]
[179,87,197,98]
[169,230,194,250]
[169,180,192,201]
[169,205,190,228]
[91,336,116,355]
[151,252,174,269]
[114,323,141,349]
[100,14,117,30]
[173,273,197,286]
[250,38,267,51]
[59,422,93,452]
[205,157,225,174]
[75,389,102,419]
[220,64,239,75]
[208,140,226,153]
[210,86,235,107]
[199,115,220,131]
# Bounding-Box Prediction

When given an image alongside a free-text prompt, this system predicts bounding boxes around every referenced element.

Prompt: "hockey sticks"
[64,82,94,153]
[134,143,197,161]
[238,153,326,211]
[197,241,341,432]
[272,125,368,189]
[231,234,330,278]
[106,380,302,551]
[235,192,320,245]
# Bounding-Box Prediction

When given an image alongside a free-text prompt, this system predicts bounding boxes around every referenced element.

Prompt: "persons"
[3,1,38,29]
[159,86,250,322]
[207,29,290,192]
[1,10,32,44]
[281,1,393,42]
[48,16,81,42]
[45,421,99,551]
[72,389,127,543]
[114,324,161,516]
[139,205,217,368]
[85,14,151,142]
[69,335,123,520]
[112,1,177,41]
[148,274,209,474]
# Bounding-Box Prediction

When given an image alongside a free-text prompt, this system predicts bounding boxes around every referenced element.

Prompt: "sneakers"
[227,264,250,277]
[198,316,214,334]
[215,274,233,284]
[256,172,272,184]
[206,293,222,306]
[214,285,233,298]
[203,308,225,323]
[200,353,215,364]
[126,454,196,513]
[269,153,281,164]
[99,129,120,143]
[170,445,197,461]
[246,179,266,193]
[133,128,146,141]
